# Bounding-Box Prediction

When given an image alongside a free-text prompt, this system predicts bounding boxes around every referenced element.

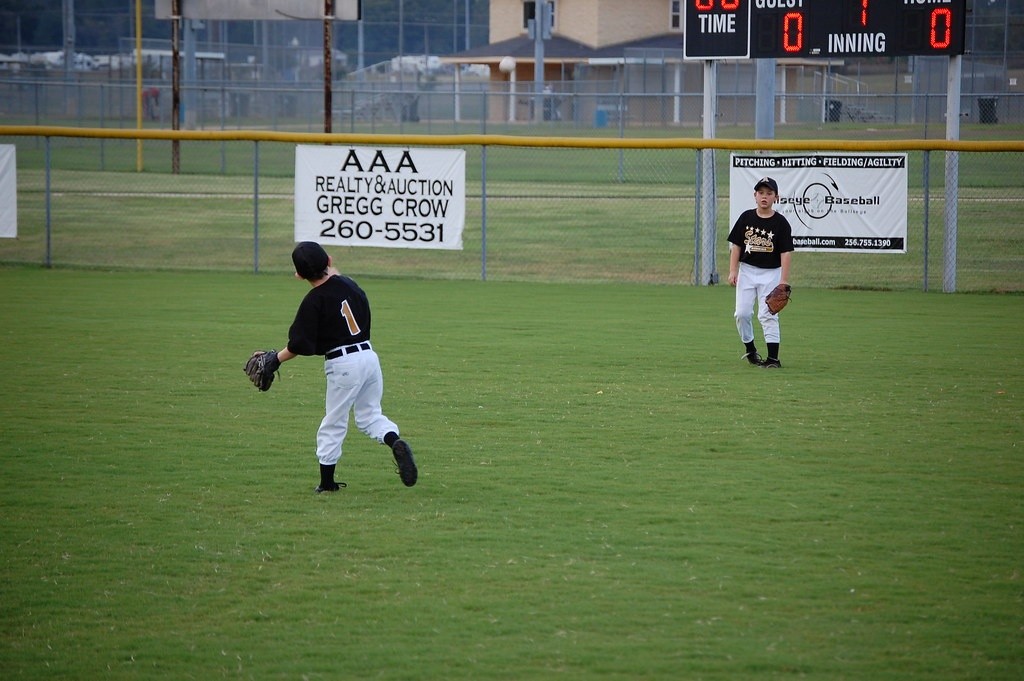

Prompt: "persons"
[243,241,419,493]
[727,177,794,368]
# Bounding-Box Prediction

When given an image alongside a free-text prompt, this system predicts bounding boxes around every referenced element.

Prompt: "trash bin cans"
[825,99,842,121]
[978,97,999,123]
[178,102,185,123]
[594,110,608,129]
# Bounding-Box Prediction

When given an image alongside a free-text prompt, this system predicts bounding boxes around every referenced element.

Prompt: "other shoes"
[765,357,781,369]
[392,439,418,488]
[742,348,766,366]
[313,479,347,494]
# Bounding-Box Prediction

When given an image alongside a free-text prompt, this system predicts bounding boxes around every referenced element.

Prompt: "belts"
[325,343,370,360]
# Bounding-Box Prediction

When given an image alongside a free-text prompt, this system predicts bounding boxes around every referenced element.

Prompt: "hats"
[754,176,778,196]
